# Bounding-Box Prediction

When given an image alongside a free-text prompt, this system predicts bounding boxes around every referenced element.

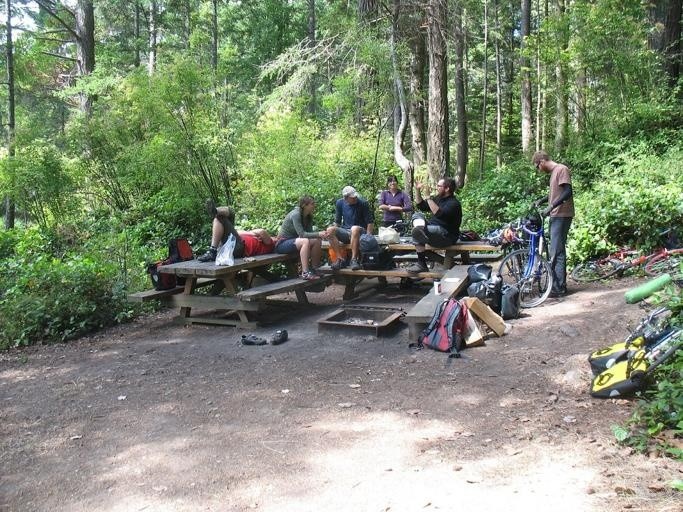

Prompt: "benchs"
[317,261,443,300]
[127,277,215,303]
[236,276,331,305]
[405,264,483,347]
[388,255,504,262]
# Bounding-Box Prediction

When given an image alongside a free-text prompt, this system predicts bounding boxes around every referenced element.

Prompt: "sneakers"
[412,228,429,243]
[197,251,215,261]
[311,270,324,276]
[207,199,216,218]
[541,288,567,297]
[351,258,360,270]
[241,335,267,346]
[330,258,347,269]
[301,272,320,279]
[270,329,288,345]
[406,263,430,273]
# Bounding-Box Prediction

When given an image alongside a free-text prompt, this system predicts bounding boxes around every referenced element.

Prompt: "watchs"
[424,197,431,202]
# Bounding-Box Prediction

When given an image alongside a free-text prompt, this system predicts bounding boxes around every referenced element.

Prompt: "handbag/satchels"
[362,250,388,271]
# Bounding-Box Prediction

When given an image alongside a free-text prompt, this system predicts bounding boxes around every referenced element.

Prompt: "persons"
[405,175,463,274]
[274,194,328,280]
[528,150,575,298]
[379,175,414,236]
[325,185,373,270]
[198,199,277,263]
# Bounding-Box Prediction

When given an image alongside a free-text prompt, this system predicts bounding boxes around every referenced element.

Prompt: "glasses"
[437,185,447,189]
[535,163,541,170]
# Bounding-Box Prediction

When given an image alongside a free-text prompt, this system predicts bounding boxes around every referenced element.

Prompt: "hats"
[342,186,359,198]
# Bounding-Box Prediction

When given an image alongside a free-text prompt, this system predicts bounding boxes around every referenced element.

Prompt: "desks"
[157,252,309,330]
[313,243,498,265]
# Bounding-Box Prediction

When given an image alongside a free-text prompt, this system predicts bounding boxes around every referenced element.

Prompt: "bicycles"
[625,305,683,374]
[571,247,683,283]
[497,208,553,308]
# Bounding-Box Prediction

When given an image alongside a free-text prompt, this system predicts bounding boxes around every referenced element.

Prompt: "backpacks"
[408,297,467,359]
